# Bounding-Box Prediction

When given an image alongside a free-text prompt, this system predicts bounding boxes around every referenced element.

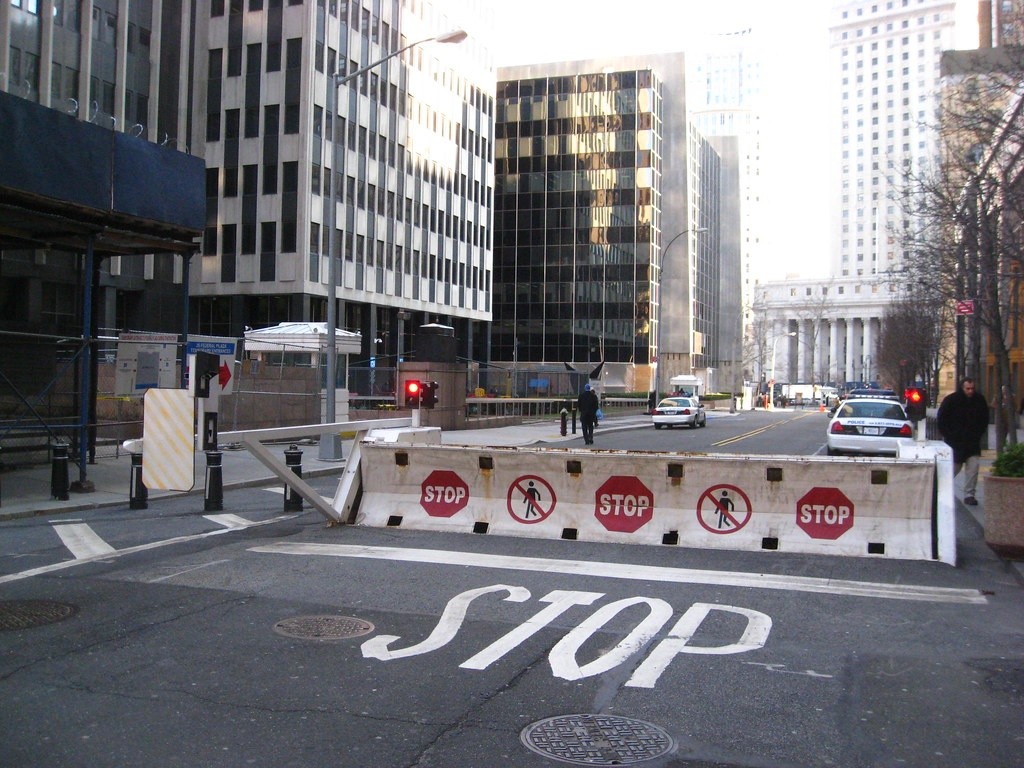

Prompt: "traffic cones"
[820,405,824,413]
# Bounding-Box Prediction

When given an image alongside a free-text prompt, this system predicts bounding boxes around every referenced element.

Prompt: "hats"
[585,384,590,390]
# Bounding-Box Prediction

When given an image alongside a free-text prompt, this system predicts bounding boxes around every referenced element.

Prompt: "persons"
[577,384,601,445]
[649,390,657,409]
[937,377,989,504]
[991,383,1021,447]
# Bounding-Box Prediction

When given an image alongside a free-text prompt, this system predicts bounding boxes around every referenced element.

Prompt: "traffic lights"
[420,382,432,407]
[907,388,923,423]
[431,380,439,407]
[404,380,419,408]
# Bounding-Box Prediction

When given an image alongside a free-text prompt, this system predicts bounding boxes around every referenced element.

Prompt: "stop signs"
[594,476,654,533]
[797,487,854,540]
[420,470,470,517]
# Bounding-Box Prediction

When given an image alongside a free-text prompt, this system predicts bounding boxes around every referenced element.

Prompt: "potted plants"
[981,440,1024,562]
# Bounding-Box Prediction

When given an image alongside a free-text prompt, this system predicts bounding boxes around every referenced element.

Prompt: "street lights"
[306,28,467,462]
[652,227,710,411]
[767,332,798,409]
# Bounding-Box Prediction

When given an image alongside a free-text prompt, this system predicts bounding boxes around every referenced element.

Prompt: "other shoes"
[595,423,599,427]
[585,440,593,444]
[964,497,977,505]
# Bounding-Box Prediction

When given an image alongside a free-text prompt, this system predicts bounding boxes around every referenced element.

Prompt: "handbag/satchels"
[596,409,604,420]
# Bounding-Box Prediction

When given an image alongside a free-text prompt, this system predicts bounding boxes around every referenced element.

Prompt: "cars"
[651,397,707,428]
[826,397,913,457]
[832,388,902,422]
